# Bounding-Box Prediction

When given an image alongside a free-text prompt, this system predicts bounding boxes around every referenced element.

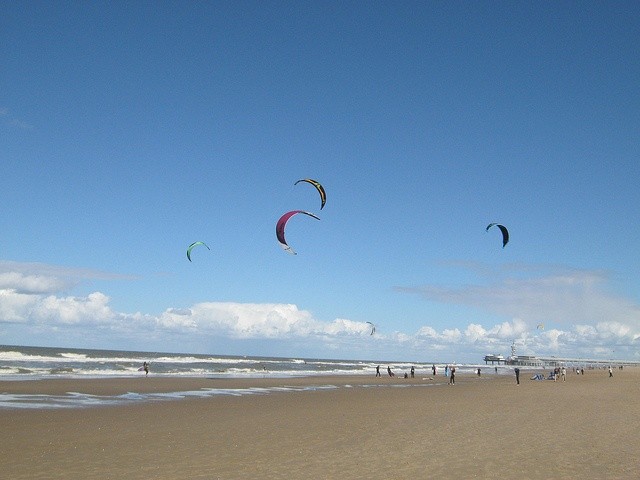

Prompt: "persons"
[376,365,381,377]
[404,373,408,378]
[387,366,395,377]
[561,367,566,382]
[619,366,624,370]
[143,361,149,375]
[477,368,481,376]
[514,369,519,384]
[445,365,449,377]
[573,366,607,375]
[450,367,455,385]
[495,365,498,374]
[411,366,414,378]
[608,365,613,377]
[432,364,436,375]
[554,368,558,381]
